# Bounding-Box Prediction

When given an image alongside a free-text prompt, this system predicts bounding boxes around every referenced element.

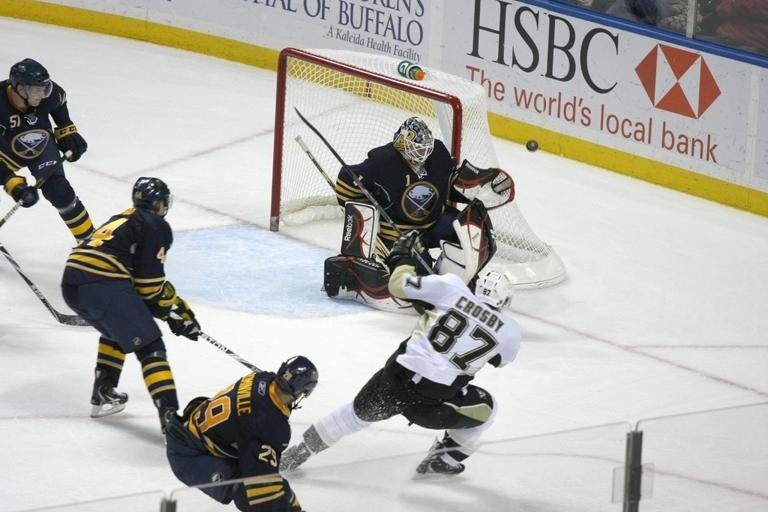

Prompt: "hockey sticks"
[295,108,436,274]
[0,244,92,326]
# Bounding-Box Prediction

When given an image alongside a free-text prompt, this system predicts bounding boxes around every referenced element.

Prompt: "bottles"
[398,60,424,80]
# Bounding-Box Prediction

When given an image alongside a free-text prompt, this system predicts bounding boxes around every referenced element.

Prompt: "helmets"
[7,57,54,108]
[474,269,514,312]
[274,356,319,409]
[132,177,171,220]
[392,117,435,164]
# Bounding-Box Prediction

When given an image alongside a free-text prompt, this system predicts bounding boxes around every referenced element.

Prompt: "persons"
[60,176,201,436]
[323,115,494,314]
[0,56,97,246]
[164,355,319,512]
[575,0,767,58]
[276,226,522,472]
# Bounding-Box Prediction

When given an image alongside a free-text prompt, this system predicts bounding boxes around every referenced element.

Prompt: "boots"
[89,368,128,418]
[277,442,310,473]
[324,255,353,299]
[414,443,466,479]
[154,398,183,445]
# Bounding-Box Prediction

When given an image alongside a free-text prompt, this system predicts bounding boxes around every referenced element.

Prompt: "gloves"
[166,307,203,341]
[53,120,87,162]
[388,230,420,263]
[3,176,39,208]
